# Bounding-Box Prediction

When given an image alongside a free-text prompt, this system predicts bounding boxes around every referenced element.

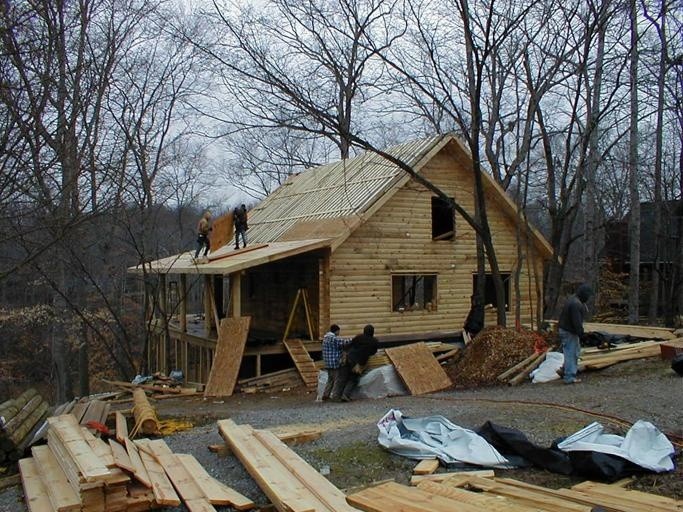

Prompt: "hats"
[330,324,340,332]
[577,284,595,296]
[364,325,374,334]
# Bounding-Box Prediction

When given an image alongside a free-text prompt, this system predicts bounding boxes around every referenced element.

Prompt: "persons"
[231,204,249,250]
[460,290,484,344]
[195,210,214,258]
[320,323,354,401]
[556,286,590,384]
[327,324,378,401]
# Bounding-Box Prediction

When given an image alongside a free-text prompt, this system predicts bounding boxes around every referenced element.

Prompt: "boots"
[243,242,246,248]
[234,243,239,249]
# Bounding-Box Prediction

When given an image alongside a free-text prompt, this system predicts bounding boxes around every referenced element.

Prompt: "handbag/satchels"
[351,363,364,374]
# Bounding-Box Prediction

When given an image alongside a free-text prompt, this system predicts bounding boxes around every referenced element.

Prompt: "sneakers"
[322,393,351,402]
[565,378,581,384]
[556,368,564,378]
